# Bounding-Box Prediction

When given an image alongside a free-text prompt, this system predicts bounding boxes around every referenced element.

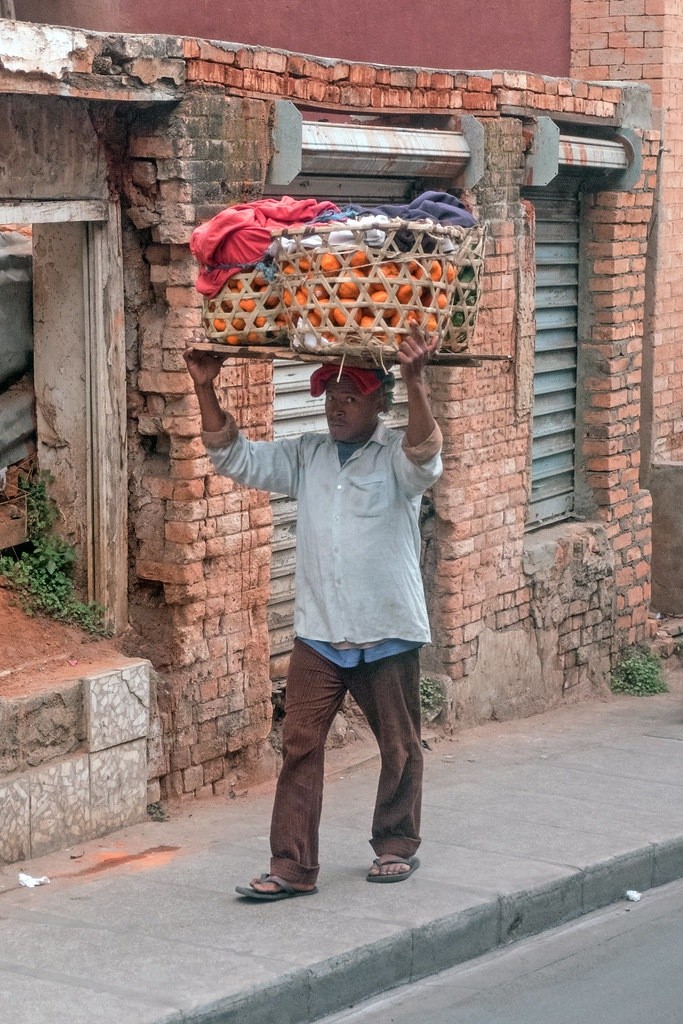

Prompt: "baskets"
[197,215,487,362]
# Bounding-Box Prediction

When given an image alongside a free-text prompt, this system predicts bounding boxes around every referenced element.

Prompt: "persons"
[183,319,442,900]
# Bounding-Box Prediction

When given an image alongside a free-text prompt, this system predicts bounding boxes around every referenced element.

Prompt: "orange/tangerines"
[201,252,455,348]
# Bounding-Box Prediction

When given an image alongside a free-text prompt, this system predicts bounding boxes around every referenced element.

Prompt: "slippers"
[366,858,420,883]
[235,873,317,900]
